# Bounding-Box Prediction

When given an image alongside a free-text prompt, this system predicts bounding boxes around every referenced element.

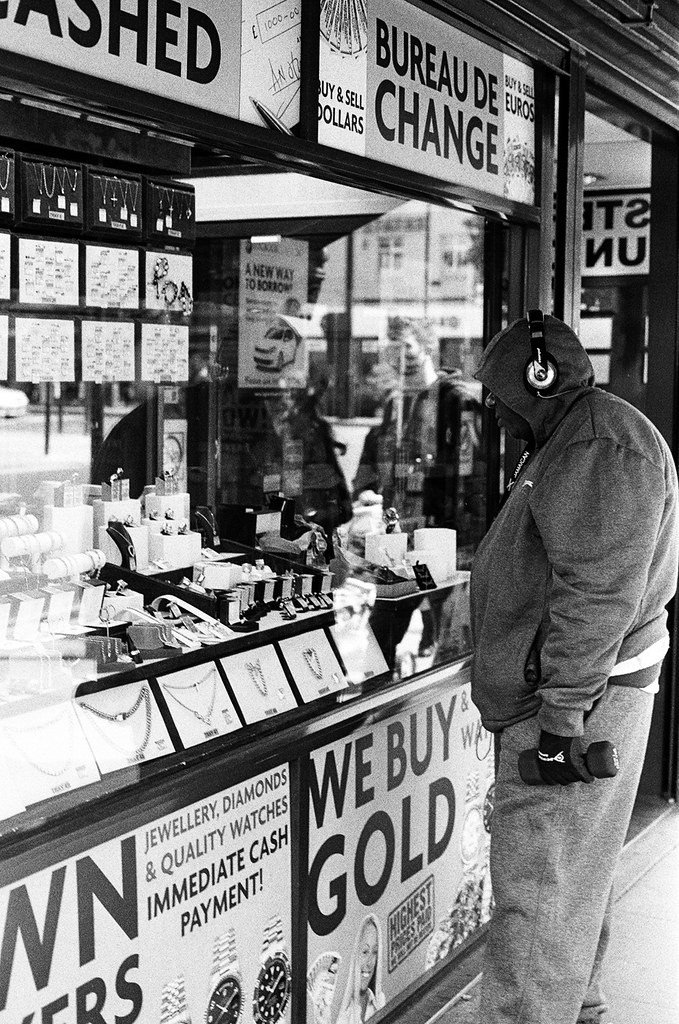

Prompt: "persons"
[464,312,679,1024]
[339,914,387,1024]
[92,315,485,550]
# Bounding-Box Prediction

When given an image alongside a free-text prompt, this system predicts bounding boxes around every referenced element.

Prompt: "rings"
[162,522,172,534]
[165,508,174,519]
[108,515,118,522]
[149,508,159,519]
[116,467,123,475]
[109,474,118,483]
[123,515,134,524]
[177,522,187,533]
[108,465,338,626]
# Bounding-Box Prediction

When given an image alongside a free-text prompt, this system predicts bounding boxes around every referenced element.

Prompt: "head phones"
[524,309,561,399]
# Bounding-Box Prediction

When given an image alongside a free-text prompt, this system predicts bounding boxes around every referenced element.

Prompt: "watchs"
[160,914,293,1024]
[455,748,496,922]
[313,532,328,555]
[384,507,397,533]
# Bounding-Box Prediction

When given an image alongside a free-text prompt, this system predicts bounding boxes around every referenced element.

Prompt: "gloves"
[536,729,595,786]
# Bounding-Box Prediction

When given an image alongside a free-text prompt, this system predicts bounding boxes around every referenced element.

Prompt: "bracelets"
[0,494,104,591]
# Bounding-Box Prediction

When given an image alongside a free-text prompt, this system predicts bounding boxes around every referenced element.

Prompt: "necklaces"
[109,525,136,557]
[9,634,330,780]
